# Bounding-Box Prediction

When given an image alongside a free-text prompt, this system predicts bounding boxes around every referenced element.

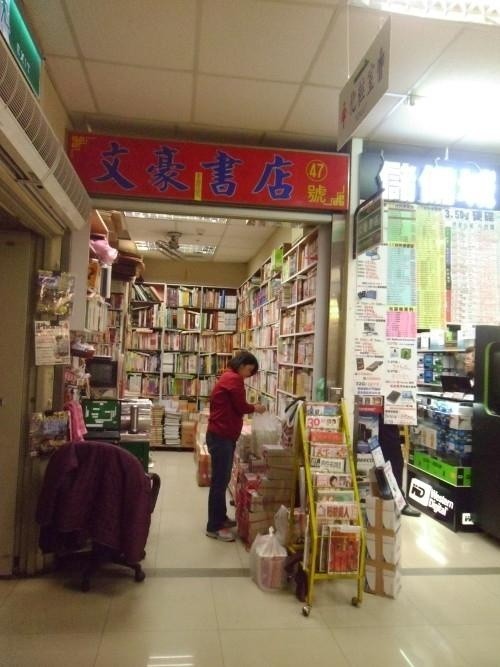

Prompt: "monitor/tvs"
[440,375,471,393]
[84,359,119,388]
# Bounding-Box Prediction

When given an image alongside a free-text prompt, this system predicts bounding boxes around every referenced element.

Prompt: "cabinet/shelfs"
[108,282,237,448]
[238,222,318,448]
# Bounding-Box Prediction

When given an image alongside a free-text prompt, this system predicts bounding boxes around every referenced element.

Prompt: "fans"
[154,231,185,262]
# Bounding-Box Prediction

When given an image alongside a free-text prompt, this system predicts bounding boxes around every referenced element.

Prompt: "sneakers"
[205,516,237,542]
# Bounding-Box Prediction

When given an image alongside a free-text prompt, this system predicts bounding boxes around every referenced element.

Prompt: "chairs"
[40,441,162,591]
